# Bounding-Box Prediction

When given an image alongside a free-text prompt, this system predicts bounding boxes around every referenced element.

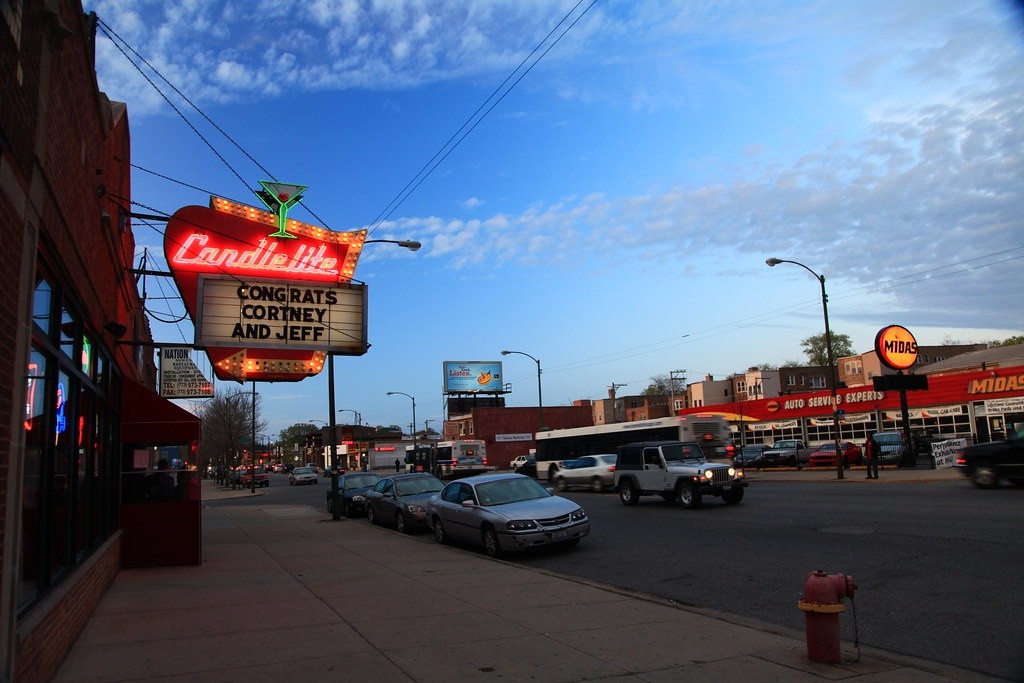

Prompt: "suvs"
[614,441,748,509]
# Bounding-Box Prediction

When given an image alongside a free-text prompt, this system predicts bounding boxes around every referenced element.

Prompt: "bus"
[404,439,486,477]
[536,412,733,483]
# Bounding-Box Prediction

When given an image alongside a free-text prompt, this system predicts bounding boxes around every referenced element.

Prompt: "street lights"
[501,351,543,427]
[225,392,259,487]
[338,409,362,470]
[765,257,846,479]
[387,392,417,472]
[329,239,421,515]
[310,420,328,467]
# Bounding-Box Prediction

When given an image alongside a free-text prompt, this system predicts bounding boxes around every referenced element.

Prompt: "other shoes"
[866,476,872,479]
[873,477,879,479]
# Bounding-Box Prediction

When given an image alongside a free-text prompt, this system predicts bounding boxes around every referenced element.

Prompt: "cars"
[734,430,914,467]
[953,424,1024,488]
[510,456,529,468]
[424,473,591,558]
[365,473,446,531]
[554,454,618,492]
[324,466,345,477]
[265,463,319,485]
[228,463,270,488]
[326,473,383,514]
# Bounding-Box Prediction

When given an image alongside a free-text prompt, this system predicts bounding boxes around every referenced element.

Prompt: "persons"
[362,463,367,472]
[122,459,190,503]
[395,458,400,472]
[864,433,879,480]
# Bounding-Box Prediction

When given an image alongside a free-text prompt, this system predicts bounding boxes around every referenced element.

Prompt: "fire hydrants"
[799,569,860,666]
